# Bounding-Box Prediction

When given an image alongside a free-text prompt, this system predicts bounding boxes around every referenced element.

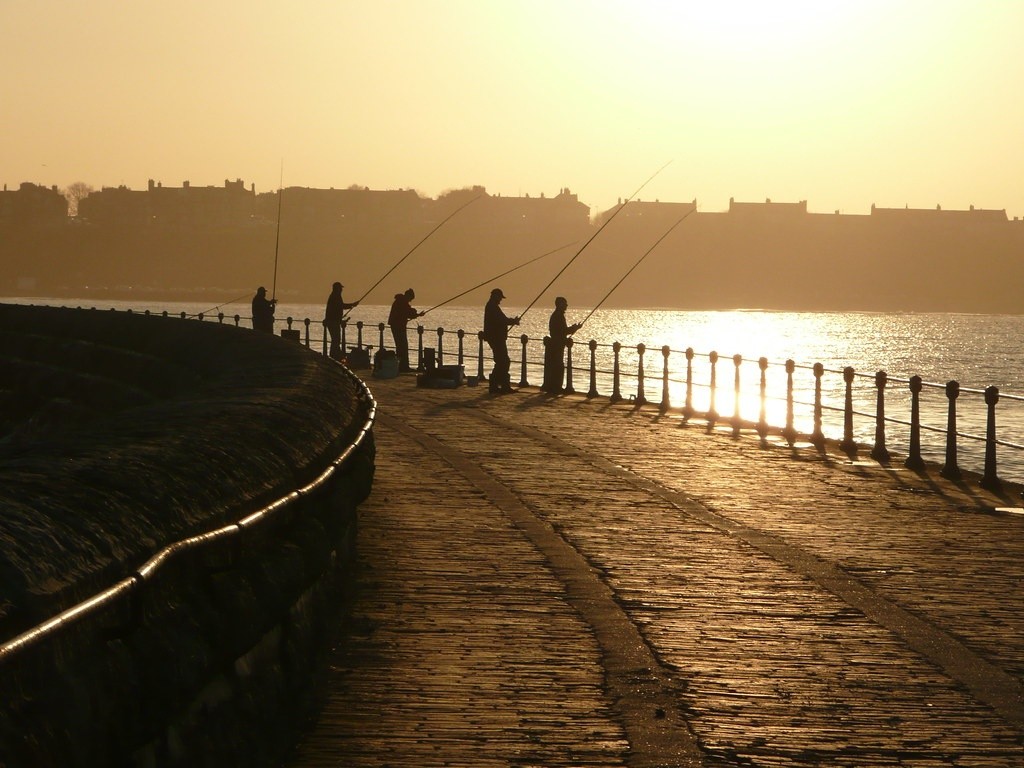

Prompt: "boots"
[489,374,499,392]
[500,373,516,394]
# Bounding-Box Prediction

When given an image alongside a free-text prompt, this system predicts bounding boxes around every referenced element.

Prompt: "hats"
[491,288,506,299]
[257,287,268,292]
[333,282,345,288]
[406,288,414,299]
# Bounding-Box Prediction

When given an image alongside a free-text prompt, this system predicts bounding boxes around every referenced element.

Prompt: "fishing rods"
[269,164,288,311]
[335,190,483,314]
[572,208,695,331]
[504,152,684,334]
[407,241,579,317]
[185,293,254,322]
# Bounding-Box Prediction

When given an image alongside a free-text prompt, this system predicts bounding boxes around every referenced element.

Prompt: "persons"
[546,297,583,392]
[388,288,424,372]
[483,288,520,393]
[325,282,359,360]
[251,287,278,332]
